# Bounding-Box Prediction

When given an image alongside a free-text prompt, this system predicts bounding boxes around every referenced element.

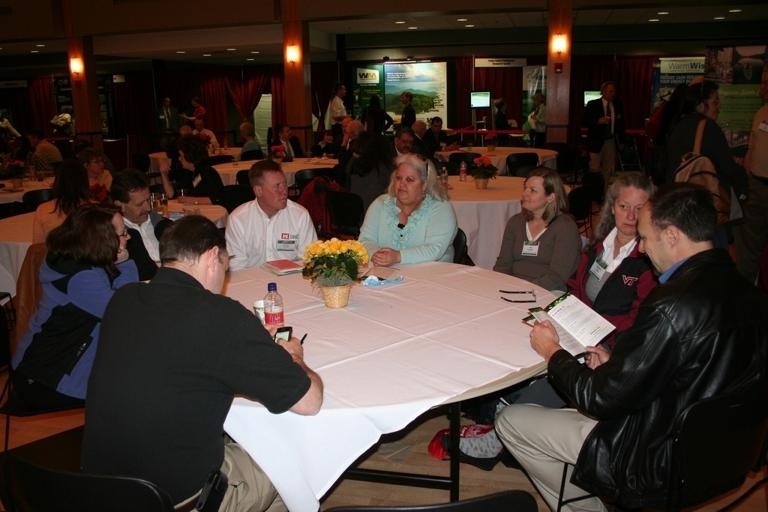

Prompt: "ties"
[583,89,603,107]
[469,91,491,109]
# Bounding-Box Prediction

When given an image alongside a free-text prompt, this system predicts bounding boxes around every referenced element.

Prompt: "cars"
[253,300,266,325]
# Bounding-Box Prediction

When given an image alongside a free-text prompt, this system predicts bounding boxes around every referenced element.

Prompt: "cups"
[499,288,536,303]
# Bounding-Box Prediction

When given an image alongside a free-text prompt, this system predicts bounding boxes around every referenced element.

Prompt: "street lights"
[294,177,342,235]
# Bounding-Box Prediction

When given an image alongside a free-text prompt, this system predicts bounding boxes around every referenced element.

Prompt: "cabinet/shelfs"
[149,193,158,211]
[264,282,284,333]
[440,166,447,189]
[53,74,111,134]
[159,189,170,214]
[459,157,467,183]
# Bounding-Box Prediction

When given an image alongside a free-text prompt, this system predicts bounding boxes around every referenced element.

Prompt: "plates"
[53,74,111,134]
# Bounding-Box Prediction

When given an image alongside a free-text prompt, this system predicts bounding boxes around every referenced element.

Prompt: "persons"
[665,81,749,261]
[72,216,322,512]
[493,167,582,292]
[224,162,319,270]
[0,107,75,177]
[492,179,762,511]
[358,155,458,267]
[240,123,264,160]
[81,149,113,200]
[147,96,225,202]
[315,84,455,234]
[33,165,99,243]
[272,124,307,162]
[494,99,521,146]
[532,94,546,147]
[439,169,666,474]
[744,82,768,186]
[109,173,172,281]
[584,81,623,189]
[11,202,138,411]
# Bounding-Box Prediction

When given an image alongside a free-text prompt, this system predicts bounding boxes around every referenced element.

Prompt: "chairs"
[0,129,586,512]
[557,372,768,512]
[569,143,603,235]
[442,436,505,470]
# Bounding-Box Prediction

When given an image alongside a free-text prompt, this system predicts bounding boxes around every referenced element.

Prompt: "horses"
[273,327,293,345]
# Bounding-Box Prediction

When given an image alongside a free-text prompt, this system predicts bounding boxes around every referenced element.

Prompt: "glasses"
[550,32,570,73]
[68,55,84,75]
[286,43,302,64]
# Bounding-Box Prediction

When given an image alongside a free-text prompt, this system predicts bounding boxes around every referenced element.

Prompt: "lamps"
[68,55,84,75]
[286,43,302,64]
[550,32,570,73]
[569,143,603,235]
[557,372,768,512]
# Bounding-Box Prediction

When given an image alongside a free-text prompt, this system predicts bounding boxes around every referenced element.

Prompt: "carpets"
[606,102,612,135]
[286,142,291,158]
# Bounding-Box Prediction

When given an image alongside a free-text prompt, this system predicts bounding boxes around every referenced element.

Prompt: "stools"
[173,497,199,511]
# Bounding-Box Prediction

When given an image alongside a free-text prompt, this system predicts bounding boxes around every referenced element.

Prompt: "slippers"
[672,120,732,225]
